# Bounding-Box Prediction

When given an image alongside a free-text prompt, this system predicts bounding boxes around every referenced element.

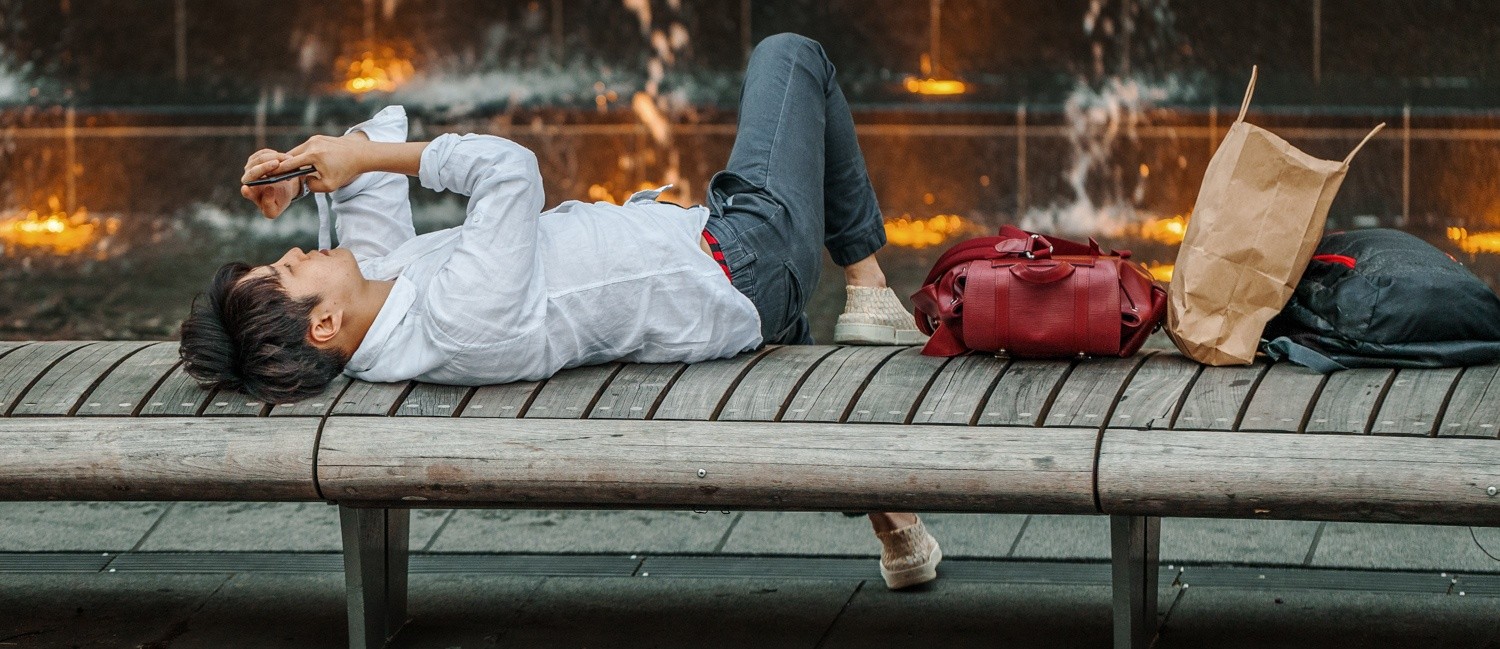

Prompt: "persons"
[180,34,883,398]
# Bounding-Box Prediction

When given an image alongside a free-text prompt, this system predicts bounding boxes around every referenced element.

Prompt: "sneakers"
[877,513,942,589]
[835,284,932,347]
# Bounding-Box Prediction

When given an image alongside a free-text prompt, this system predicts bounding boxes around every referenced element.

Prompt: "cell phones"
[244,168,316,186]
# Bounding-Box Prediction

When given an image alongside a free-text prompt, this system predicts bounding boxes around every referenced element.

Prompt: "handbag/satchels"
[909,225,1169,359]
[1163,66,1386,367]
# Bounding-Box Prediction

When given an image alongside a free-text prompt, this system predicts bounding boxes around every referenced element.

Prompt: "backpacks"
[1256,228,1500,373]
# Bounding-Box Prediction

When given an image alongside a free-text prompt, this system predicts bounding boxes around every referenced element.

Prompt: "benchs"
[0,339,1500,649]
